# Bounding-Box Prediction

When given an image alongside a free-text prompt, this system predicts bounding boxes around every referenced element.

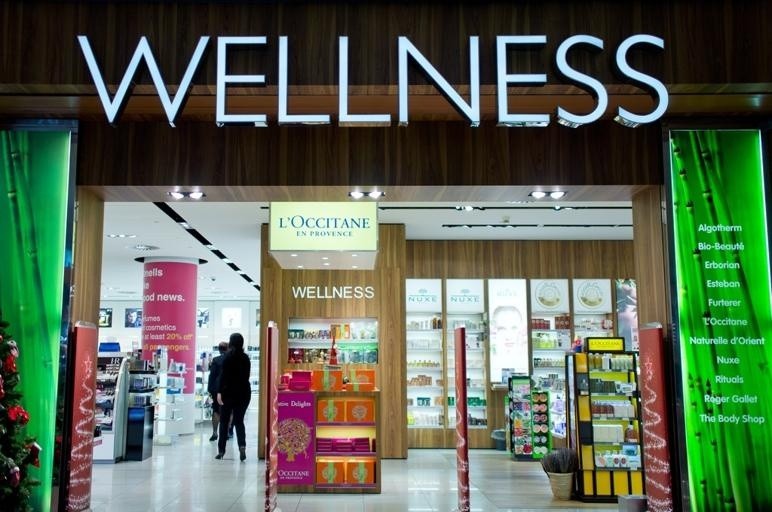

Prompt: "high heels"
[215,451,225,460]
[239,447,246,461]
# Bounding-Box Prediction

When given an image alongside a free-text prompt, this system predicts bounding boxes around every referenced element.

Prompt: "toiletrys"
[408,360,439,367]
[288,323,350,364]
[421,317,442,330]
[468,414,487,425]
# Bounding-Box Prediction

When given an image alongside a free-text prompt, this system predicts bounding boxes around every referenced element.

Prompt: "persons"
[126,309,137,327]
[492,306,523,351]
[214,333,251,461]
[208,341,233,440]
[200,351,211,393]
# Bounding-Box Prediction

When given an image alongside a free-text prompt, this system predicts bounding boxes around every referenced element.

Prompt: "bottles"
[555,396,561,412]
[625,425,637,442]
[344,324,351,338]
[554,418,566,433]
[590,379,636,393]
[592,400,635,419]
[330,346,337,364]
[588,353,632,371]
[534,315,568,329]
[534,357,564,367]
[537,374,564,387]
[532,333,563,348]
[595,449,630,469]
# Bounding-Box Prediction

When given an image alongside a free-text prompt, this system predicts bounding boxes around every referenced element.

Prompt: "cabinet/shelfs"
[288,317,381,365]
[571,276,614,342]
[588,353,642,473]
[528,271,579,441]
[405,276,446,447]
[153,354,186,445]
[93,352,125,464]
[127,360,155,410]
[445,279,489,446]
[276,385,379,494]
[196,355,211,423]
